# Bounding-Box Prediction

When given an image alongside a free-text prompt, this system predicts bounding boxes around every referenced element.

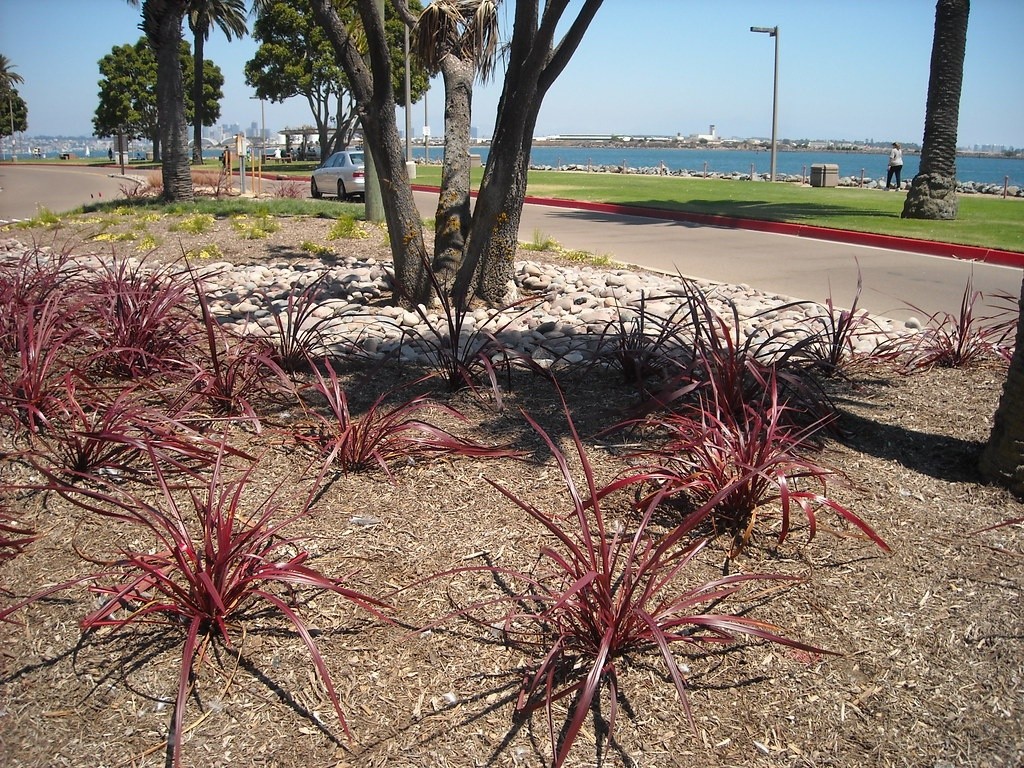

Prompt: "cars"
[311,149,366,201]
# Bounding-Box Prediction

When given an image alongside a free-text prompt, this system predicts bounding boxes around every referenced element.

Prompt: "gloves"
[888,165,891,171]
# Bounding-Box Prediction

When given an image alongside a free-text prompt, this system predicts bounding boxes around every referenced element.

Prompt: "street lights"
[749,25,780,184]
[249,96,267,165]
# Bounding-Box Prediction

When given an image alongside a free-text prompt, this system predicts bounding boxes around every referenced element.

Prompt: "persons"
[291,146,315,161]
[246,146,251,162]
[137,153,145,160]
[108,148,112,161]
[222,145,230,169]
[883,142,904,192]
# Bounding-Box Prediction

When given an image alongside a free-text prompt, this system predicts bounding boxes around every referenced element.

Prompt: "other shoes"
[895,188,900,192]
[882,187,890,191]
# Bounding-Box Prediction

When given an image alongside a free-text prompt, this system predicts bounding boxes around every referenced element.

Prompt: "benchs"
[267,157,291,160]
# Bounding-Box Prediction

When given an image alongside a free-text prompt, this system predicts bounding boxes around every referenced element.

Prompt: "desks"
[262,153,292,164]
